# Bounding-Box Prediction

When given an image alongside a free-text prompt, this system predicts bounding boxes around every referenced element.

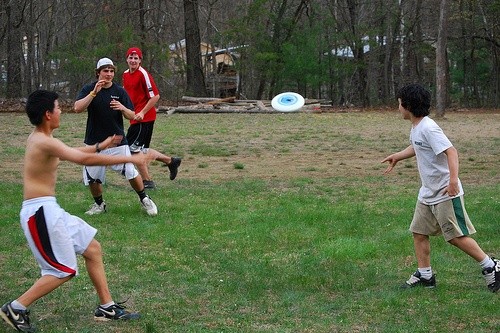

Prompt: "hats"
[126,48,143,60]
[96,57,114,70]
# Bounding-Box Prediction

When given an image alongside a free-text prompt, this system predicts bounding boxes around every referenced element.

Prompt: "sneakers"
[94,296,142,321]
[84,199,107,216]
[162,156,181,180]
[399,269,437,291]
[482,257,500,293]
[132,179,156,191]
[140,194,157,216]
[0,300,35,333]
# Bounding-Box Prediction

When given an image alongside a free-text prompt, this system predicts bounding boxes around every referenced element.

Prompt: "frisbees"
[270,91,305,112]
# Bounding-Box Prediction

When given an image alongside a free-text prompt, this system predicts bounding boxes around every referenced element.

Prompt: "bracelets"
[90,92,96,97]
[96,142,101,152]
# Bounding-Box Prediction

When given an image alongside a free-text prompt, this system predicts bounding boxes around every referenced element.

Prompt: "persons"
[0,90,158,333]
[122,47,181,189]
[381,85,500,292]
[74,57,158,216]
